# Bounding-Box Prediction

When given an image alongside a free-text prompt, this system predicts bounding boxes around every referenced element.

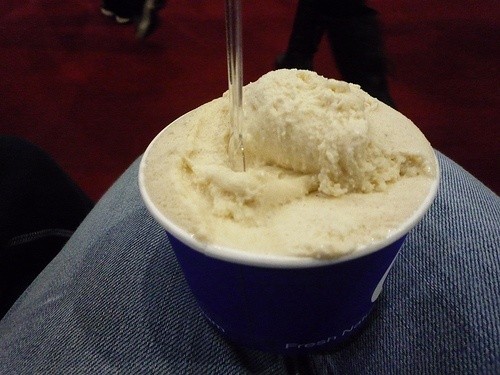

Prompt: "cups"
[138,105,444,352]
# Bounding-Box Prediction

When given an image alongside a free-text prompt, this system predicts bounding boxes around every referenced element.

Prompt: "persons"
[0,135,93,321]
[274,0,397,109]
[101,0,166,41]
[0,148,500,375]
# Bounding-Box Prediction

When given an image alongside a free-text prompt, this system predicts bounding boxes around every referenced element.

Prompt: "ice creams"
[143,66,432,260]
